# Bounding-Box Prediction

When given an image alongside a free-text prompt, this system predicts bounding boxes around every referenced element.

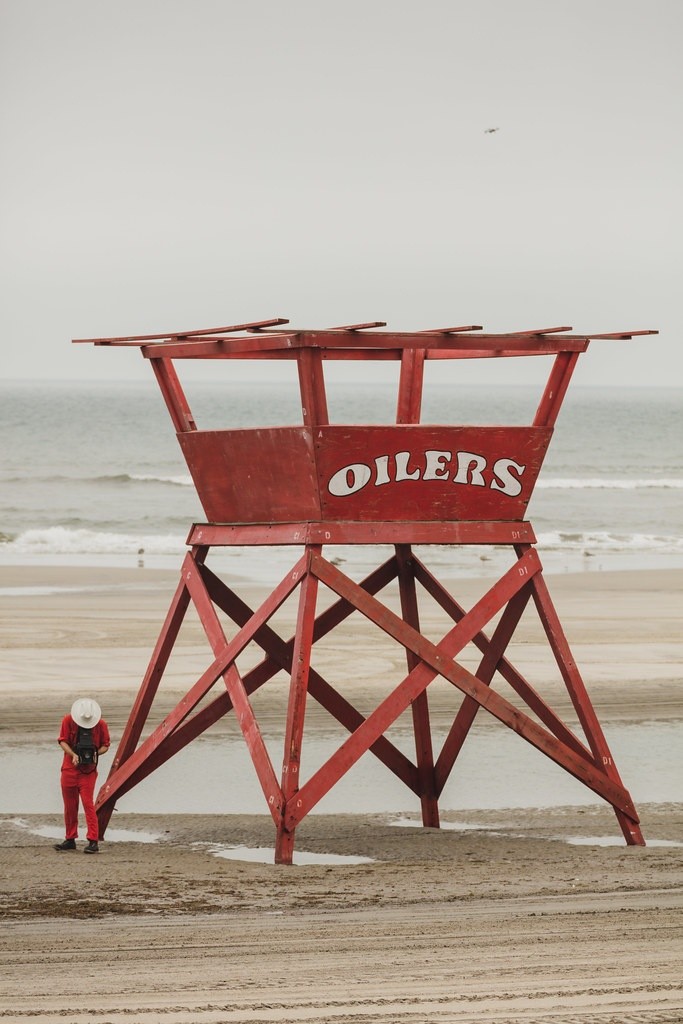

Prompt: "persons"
[52,698,110,854]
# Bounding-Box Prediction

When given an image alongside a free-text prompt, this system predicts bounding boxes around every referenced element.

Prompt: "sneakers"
[84,841,98,853]
[52,840,76,850]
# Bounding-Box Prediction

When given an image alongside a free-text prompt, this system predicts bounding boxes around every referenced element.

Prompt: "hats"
[71,699,101,728]
